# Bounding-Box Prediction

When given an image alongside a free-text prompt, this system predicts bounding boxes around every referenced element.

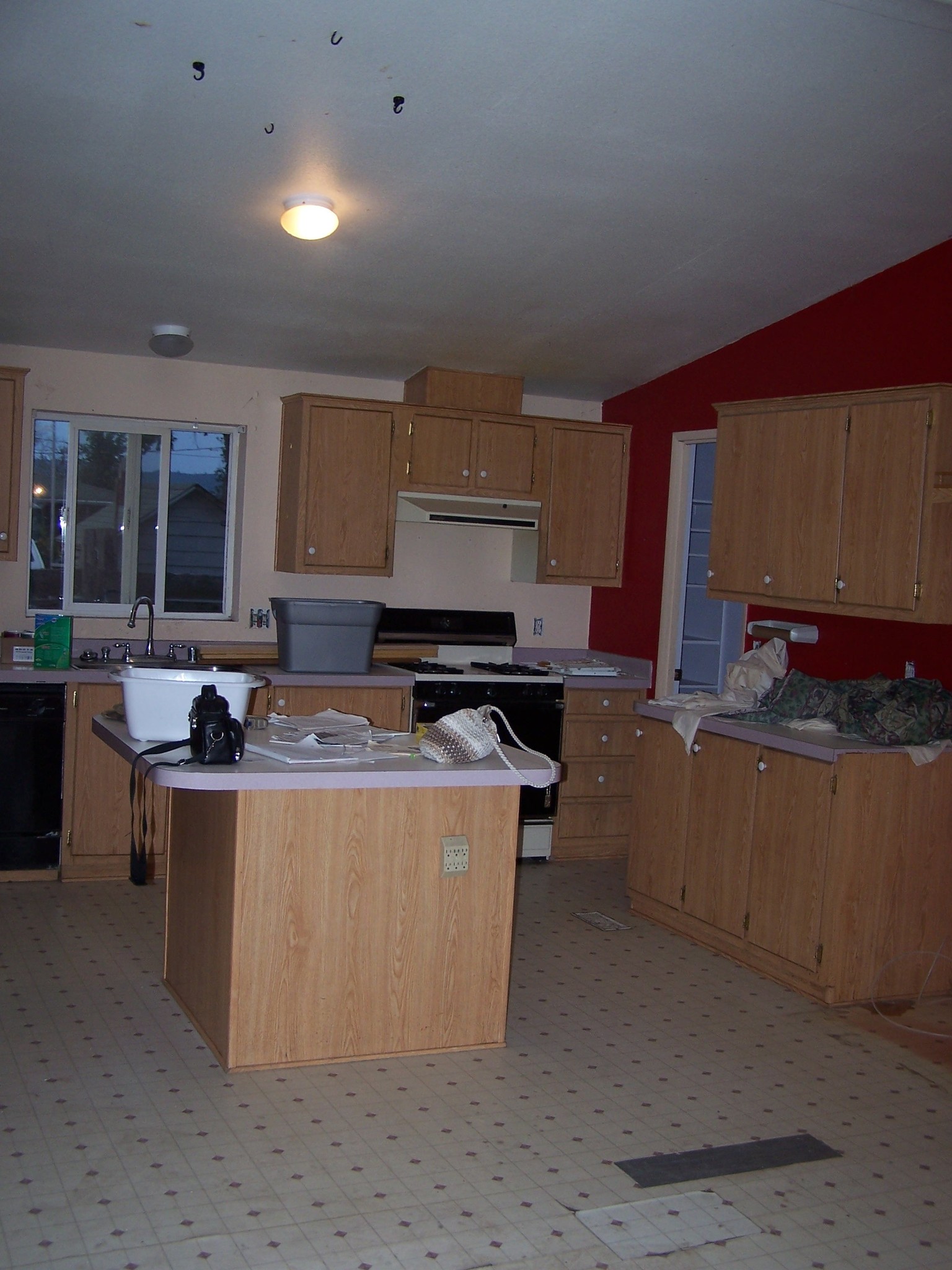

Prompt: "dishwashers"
[0,682,67,871]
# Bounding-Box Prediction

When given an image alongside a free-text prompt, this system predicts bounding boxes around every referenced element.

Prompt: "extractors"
[395,491,541,530]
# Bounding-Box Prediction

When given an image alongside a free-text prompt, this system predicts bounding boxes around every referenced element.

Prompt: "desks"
[93,710,564,1074]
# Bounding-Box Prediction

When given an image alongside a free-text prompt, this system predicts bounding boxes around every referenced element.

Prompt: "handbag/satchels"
[420,709,499,763]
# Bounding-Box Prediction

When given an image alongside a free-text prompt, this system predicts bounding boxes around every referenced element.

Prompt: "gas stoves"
[377,607,566,683]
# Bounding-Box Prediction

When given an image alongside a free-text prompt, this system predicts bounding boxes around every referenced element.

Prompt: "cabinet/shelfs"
[623,713,952,1008]
[63,684,411,880]
[512,424,632,589]
[551,688,643,863]
[273,400,398,578]
[396,409,539,502]
[0,365,30,562]
[705,383,952,626]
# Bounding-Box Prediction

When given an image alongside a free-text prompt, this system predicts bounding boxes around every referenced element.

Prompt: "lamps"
[280,195,340,240]
[148,325,193,359]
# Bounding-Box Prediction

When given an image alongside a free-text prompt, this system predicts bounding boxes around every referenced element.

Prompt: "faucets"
[126,596,156,662]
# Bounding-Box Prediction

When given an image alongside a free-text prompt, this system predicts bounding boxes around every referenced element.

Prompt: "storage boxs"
[0,637,35,663]
[107,668,264,743]
[415,722,432,745]
[34,613,75,669]
[268,597,387,675]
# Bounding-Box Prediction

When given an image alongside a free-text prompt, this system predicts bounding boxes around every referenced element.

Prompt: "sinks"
[71,662,249,673]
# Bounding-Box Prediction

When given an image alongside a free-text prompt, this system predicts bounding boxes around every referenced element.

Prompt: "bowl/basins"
[107,667,266,743]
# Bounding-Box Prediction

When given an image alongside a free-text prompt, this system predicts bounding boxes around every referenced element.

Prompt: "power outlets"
[533,618,543,638]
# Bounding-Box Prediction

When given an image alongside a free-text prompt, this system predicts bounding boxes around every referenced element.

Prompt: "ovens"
[410,682,565,822]
